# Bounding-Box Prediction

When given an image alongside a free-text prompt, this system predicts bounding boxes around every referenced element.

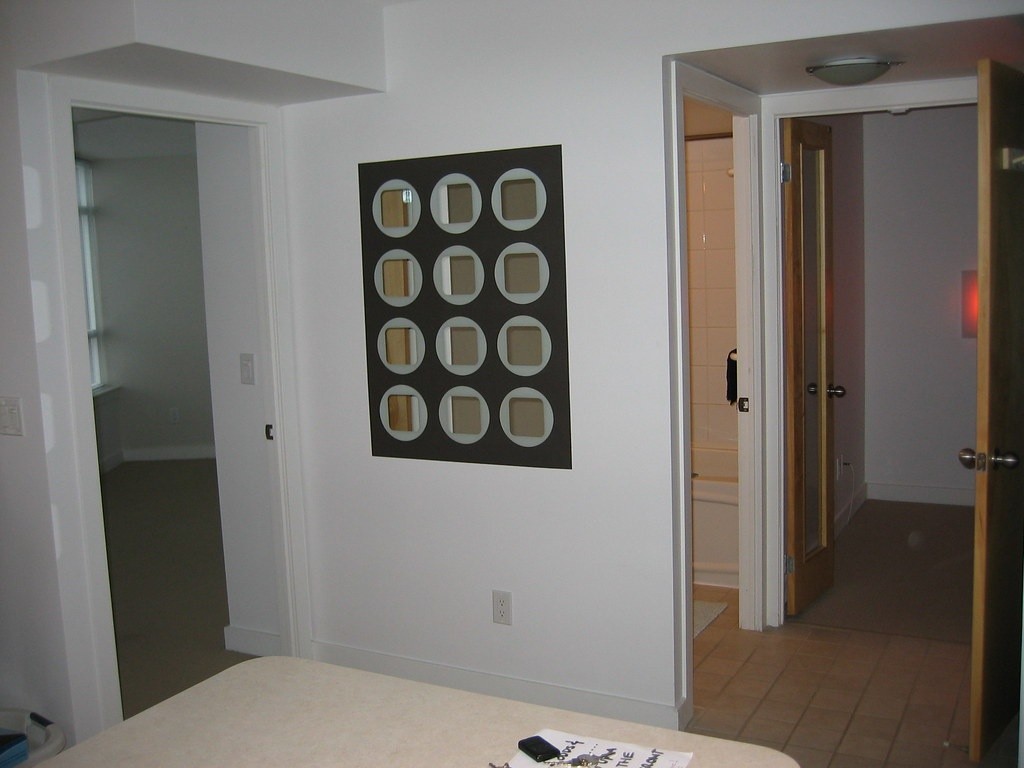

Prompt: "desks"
[33,654,805,768]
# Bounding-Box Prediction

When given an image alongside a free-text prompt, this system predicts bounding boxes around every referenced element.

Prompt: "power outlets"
[492,590,513,625]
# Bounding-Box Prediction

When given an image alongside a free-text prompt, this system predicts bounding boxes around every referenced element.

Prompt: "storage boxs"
[0,708,67,768]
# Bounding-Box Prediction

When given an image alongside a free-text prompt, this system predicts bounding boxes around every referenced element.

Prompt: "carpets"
[693,600,729,637]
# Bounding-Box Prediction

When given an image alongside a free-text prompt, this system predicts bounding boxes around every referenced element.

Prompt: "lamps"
[803,57,894,88]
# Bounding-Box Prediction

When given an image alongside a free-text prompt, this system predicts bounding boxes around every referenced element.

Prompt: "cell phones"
[518,736,560,762]
